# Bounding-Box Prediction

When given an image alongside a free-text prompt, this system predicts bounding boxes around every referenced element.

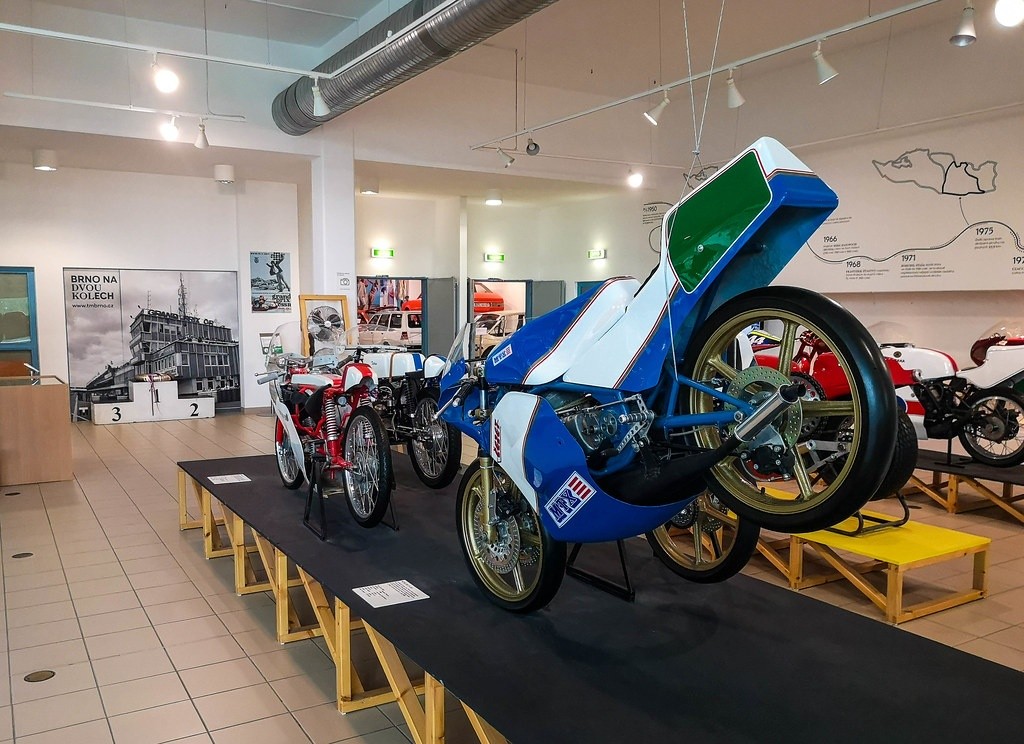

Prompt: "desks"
[810,449,1024,524]
[669,485,992,624]
[177,450,1024,744]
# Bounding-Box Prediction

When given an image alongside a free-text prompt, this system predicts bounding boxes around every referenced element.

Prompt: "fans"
[307,306,344,356]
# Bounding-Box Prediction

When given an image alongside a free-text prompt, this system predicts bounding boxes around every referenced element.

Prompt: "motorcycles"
[338,324,462,490]
[422,137,1024,617]
[255,319,394,528]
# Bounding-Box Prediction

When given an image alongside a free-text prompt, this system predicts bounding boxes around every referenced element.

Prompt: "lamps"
[525,138,540,157]
[725,77,745,109]
[213,164,236,184]
[496,147,516,168]
[643,98,670,127]
[811,50,839,86]
[485,189,503,206]
[360,178,380,195]
[948,0,976,48]
[194,123,211,150]
[311,85,331,116]
[32,148,60,171]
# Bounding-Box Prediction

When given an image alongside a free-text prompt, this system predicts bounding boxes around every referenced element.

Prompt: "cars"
[401,283,505,321]
[358,311,422,349]
[474,310,525,357]
